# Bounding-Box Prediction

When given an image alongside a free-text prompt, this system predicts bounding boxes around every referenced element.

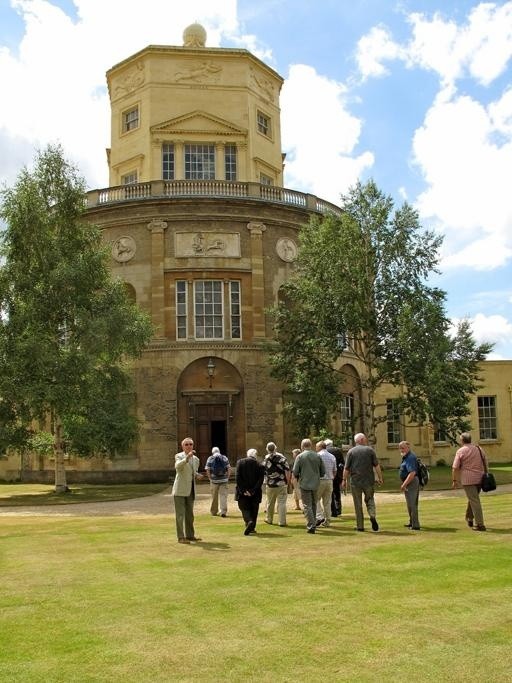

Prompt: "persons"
[204,446,232,517]
[340,432,383,531]
[315,440,338,526]
[398,440,421,529]
[292,438,326,533]
[234,448,266,535]
[262,441,291,527]
[172,436,204,544]
[451,431,489,532]
[323,438,345,517]
[291,447,302,510]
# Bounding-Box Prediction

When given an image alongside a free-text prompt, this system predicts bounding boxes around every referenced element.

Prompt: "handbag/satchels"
[481,473,496,492]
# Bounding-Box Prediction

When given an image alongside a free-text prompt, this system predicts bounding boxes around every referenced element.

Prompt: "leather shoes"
[370,517,378,531]
[178,536,202,543]
[404,524,419,530]
[244,507,341,535]
[468,520,485,531]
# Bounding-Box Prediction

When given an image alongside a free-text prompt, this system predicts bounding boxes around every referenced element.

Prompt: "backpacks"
[212,457,225,476]
[417,458,429,486]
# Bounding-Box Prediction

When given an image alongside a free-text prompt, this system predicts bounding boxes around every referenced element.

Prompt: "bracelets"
[453,479,456,481]
[341,478,346,481]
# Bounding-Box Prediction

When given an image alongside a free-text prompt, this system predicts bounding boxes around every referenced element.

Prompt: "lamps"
[206,358,216,388]
